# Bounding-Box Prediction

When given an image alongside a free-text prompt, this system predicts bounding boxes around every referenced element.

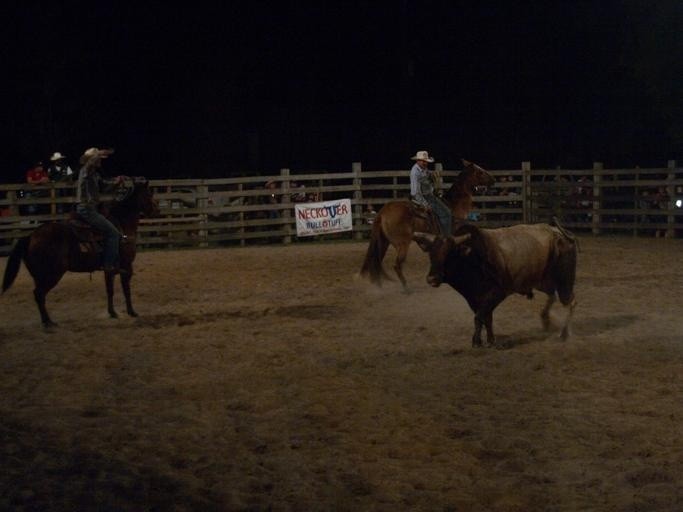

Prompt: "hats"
[411,151,434,163]
[50,153,66,161]
[80,148,107,164]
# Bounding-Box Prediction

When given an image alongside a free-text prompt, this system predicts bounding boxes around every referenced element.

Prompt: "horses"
[356,157,498,296]
[1,174,162,326]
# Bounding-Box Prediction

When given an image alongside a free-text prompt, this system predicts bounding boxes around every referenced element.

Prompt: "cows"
[411,222,579,349]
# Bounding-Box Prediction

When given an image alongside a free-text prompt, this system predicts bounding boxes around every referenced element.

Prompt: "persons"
[477,173,682,240]
[409,151,452,235]
[0,153,74,256]
[364,204,377,224]
[76,148,123,280]
[149,180,317,244]
